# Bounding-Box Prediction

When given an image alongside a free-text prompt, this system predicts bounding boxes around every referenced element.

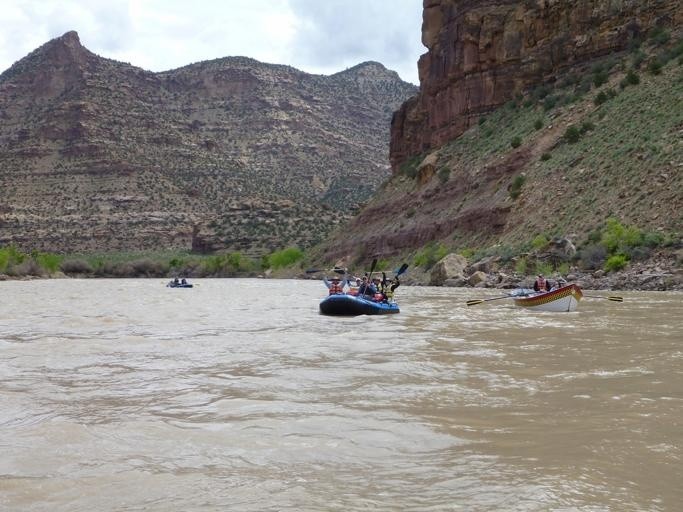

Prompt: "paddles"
[466,291,624,307]
[387,264,408,289]
[306,267,363,282]
[363,258,377,298]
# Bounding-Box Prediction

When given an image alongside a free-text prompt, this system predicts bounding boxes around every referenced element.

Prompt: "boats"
[320,294,399,315]
[514,284,583,311]
[169,281,192,288]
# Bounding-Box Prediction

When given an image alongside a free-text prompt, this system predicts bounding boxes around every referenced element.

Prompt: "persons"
[533,274,551,292]
[555,273,567,289]
[346,271,400,307]
[174,275,179,282]
[322,268,347,297]
[182,279,186,284]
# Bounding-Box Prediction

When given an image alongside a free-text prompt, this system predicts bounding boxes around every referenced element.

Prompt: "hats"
[355,278,361,281]
[331,276,339,281]
